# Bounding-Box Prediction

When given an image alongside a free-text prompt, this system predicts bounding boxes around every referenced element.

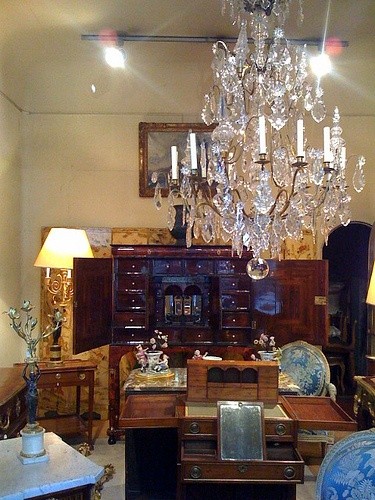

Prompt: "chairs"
[314,428,375,500]
[271,340,337,401]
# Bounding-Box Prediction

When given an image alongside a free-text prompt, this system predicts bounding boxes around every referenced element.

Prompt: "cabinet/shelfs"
[74,256,328,446]
[1,368,31,440]
[123,367,292,500]
[13,360,97,449]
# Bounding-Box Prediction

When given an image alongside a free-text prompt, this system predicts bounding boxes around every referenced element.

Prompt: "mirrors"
[219,404,263,462]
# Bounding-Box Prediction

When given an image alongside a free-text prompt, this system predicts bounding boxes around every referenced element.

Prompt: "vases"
[257,351,276,361]
[145,352,163,369]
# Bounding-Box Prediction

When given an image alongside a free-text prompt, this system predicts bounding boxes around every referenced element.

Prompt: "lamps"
[80,34,349,79]
[366,259,375,305]
[33,227,94,318]
[151,1,369,279]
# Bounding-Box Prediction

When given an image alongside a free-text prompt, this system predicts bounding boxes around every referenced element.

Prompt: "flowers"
[145,329,168,350]
[253,333,277,352]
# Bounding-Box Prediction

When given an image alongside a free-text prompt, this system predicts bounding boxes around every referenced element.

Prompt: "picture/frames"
[138,121,228,198]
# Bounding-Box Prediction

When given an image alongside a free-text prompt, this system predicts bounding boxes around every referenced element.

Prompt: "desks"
[0,432,104,500]
[354,376,375,433]
